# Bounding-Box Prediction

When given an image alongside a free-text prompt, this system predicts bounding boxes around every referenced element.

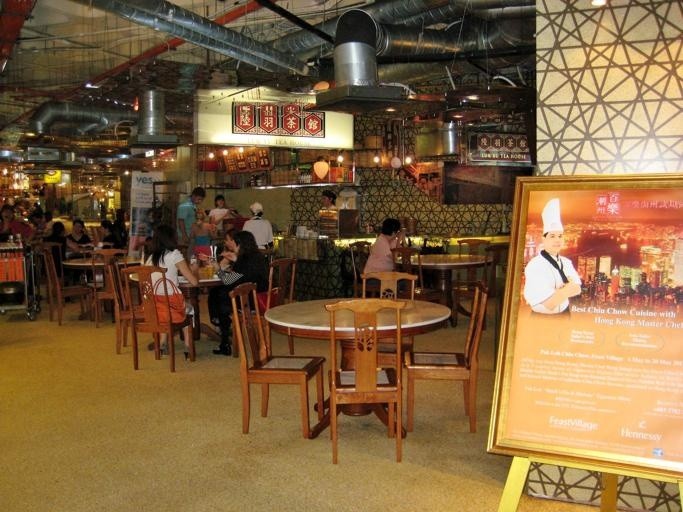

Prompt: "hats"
[250,202,264,215]
[322,190,337,200]
[541,198,564,237]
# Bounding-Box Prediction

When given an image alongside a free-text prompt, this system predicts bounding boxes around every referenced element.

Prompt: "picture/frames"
[484,172,683,485]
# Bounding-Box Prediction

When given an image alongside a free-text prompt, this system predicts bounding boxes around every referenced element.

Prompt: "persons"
[363,218,408,298]
[142,187,274,361]
[1,198,127,286]
[523,229,582,319]
[319,189,337,211]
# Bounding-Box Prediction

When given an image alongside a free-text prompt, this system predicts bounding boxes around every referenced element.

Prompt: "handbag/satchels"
[144,279,186,323]
[255,286,281,315]
[217,264,244,285]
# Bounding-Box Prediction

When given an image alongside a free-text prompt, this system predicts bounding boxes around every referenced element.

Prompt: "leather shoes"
[213,346,231,356]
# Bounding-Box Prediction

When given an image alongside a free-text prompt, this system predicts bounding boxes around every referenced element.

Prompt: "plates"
[295,225,318,240]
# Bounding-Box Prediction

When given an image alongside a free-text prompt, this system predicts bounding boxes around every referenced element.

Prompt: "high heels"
[183,352,189,361]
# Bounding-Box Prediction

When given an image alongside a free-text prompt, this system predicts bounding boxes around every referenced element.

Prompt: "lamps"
[406,125,412,164]
[373,125,380,163]
